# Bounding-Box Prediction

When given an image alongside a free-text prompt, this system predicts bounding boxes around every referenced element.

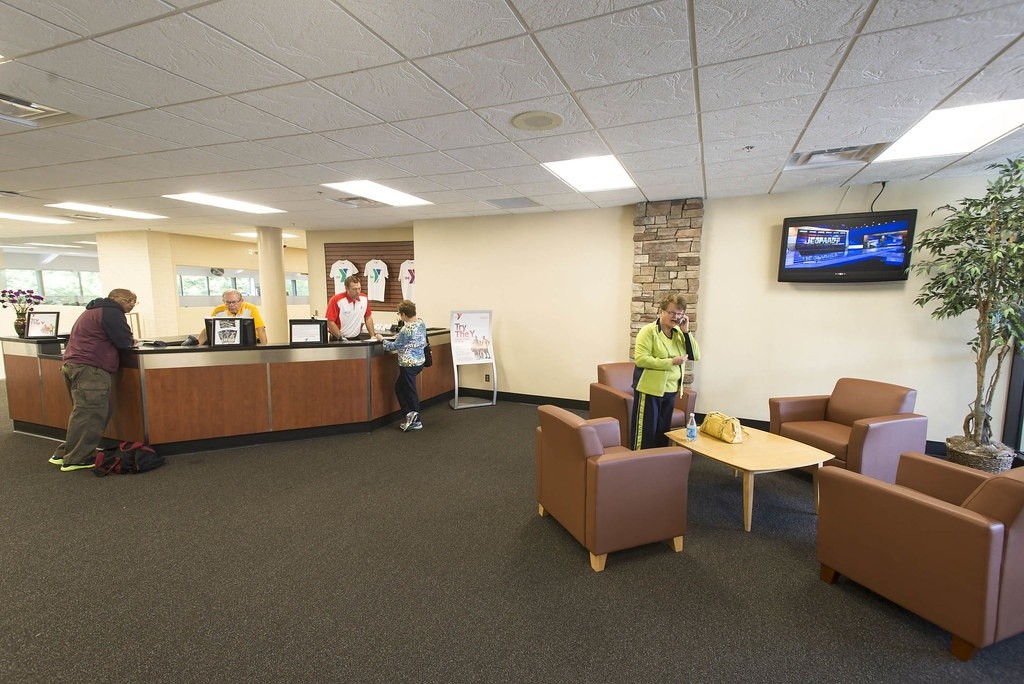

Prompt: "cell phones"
[677,317,684,325]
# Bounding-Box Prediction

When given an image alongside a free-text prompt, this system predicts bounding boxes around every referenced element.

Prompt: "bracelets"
[382,339,384,343]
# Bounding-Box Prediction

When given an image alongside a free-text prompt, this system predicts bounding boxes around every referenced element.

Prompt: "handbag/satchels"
[699,410,749,444]
[424,332,432,366]
[93,440,165,477]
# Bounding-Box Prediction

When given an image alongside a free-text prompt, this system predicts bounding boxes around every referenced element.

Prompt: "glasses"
[397,311,401,315]
[121,297,139,305]
[223,298,241,304]
[665,309,683,316]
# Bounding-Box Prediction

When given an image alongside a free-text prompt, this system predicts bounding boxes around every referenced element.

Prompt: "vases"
[13,313,26,338]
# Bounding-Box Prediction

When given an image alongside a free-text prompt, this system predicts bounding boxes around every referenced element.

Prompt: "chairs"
[768,377,928,483]
[535,403,693,574]
[588,361,697,449]
[816,452,1024,663]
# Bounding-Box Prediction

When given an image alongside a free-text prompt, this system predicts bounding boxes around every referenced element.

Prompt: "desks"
[664,424,836,534]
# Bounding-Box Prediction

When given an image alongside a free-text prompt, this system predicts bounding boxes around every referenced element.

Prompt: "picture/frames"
[25,312,60,339]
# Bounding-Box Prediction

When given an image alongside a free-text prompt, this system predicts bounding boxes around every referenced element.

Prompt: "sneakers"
[400,421,423,429]
[60,457,97,471]
[404,410,419,432]
[48,454,64,464]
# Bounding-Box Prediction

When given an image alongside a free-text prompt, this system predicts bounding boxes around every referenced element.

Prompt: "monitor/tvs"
[205,318,256,347]
[289,318,328,346]
[778,209,918,283]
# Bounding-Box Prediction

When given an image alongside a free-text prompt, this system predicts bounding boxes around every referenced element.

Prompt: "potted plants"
[902,153,1024,474]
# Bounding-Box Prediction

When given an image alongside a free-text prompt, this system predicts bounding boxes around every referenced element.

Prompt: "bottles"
[686,413,697,442]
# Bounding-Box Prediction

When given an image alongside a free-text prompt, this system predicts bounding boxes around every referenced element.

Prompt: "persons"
[630,293,700,451]
[197,289,268,346]
[375,300,427,432]
[325,276,375,341]
[49,288,139,472]
[50,324,54,335]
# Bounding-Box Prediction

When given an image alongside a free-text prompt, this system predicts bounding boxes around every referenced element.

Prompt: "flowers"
[0,289,44,314]
